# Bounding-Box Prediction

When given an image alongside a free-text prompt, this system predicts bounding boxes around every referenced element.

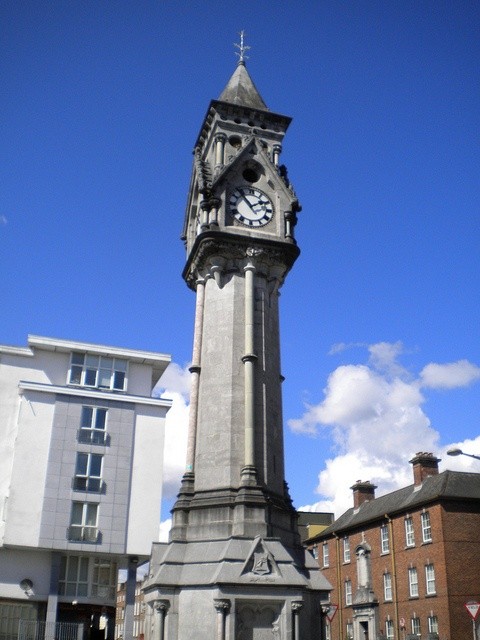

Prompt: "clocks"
[227,186,275,227]
